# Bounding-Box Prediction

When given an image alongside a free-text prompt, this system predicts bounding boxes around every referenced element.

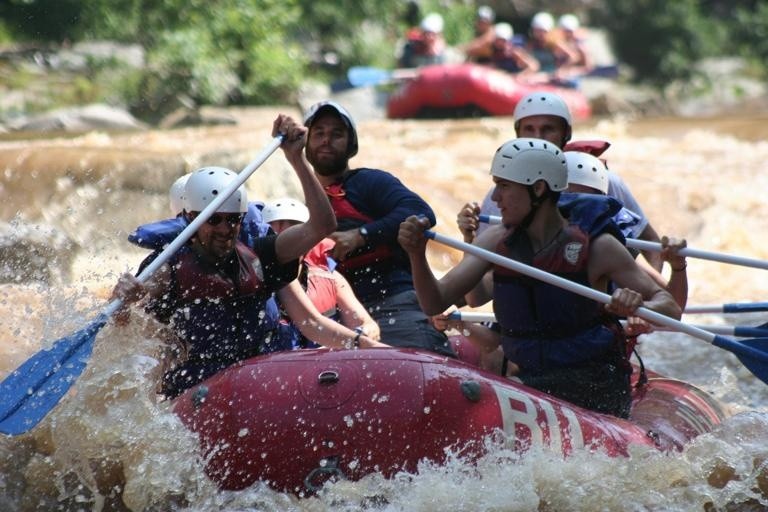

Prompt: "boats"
[386,67,590,130]
[173,335,726,498]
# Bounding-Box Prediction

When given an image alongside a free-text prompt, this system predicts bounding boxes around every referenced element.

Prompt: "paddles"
[583,65,618,78]
[1,134,287,435]
[348,66,416,86]
[423,230,768,382]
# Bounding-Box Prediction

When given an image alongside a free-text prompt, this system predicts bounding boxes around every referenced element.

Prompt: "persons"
[457,150,687,359]
[262,198,380,350]
[398,137,680,419]
[477,92,663,375]
[108,116,338,396]
[305,101,456,358]
[402,6,594,84]
[136,173,390,349]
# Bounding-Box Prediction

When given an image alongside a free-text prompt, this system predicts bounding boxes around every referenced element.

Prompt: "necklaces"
[532,228,564,255]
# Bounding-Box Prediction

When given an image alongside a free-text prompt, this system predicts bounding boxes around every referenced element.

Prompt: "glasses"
[207,213,240,226]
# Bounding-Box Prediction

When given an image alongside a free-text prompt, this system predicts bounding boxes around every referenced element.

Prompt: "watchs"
[360,225,368,250]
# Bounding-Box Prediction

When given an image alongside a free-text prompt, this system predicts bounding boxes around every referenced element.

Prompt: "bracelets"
[672,263,686,272]
[353,334,359,350]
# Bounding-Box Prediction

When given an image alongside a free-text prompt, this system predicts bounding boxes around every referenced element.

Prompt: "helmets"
[422,7,579,40]
[303,100,358,159]
[489,137,569,192]
[513,92,572,141]
[183,166,248,214]
[564,151,610,195]
[169,172,192,215]
[262,198,310,223]
